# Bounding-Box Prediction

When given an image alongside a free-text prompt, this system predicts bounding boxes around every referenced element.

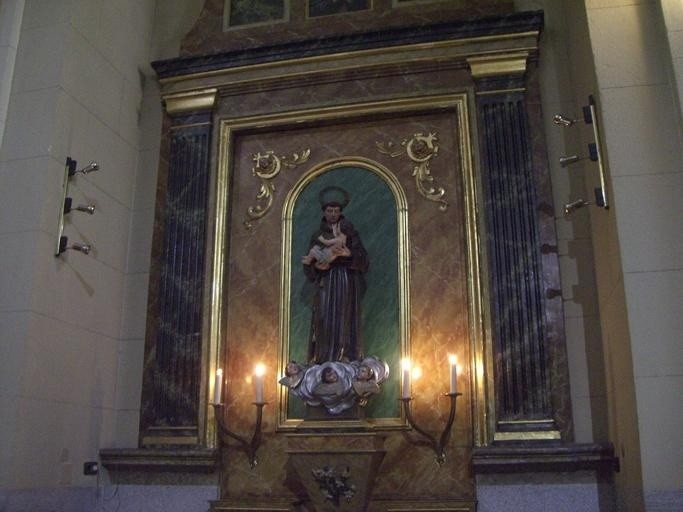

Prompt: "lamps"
[551,102,606,215]
[56,151,99,256]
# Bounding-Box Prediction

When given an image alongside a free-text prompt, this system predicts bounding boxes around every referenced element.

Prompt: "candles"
[213,367,222,404]
[401,358,410,398]
[254,363,262,403]
[449,356,456,393]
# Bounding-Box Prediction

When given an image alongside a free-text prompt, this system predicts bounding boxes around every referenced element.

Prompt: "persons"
[276,360,306,390]
[312,366,345,398]
[301,218,354,268]
[350,364,382,397]
[297,201,369,367]
